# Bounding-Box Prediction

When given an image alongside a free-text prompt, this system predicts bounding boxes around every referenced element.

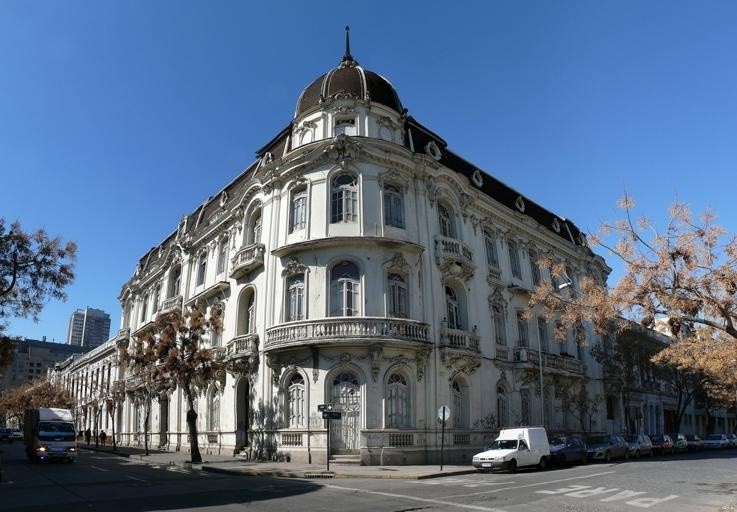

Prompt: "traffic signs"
[323,411,342,419]
[317,404,333,411]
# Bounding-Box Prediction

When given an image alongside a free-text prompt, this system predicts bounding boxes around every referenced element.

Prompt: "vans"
[471,427,551,473]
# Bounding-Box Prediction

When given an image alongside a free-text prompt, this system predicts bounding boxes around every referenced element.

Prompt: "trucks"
[23,407,78,462]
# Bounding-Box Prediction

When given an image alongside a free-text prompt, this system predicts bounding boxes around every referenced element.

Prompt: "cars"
[550,432,737,468]
[0,427,24,444]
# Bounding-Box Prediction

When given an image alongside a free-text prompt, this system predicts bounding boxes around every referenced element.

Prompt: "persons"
[97,429,107,447]
[84,427,92,446]
